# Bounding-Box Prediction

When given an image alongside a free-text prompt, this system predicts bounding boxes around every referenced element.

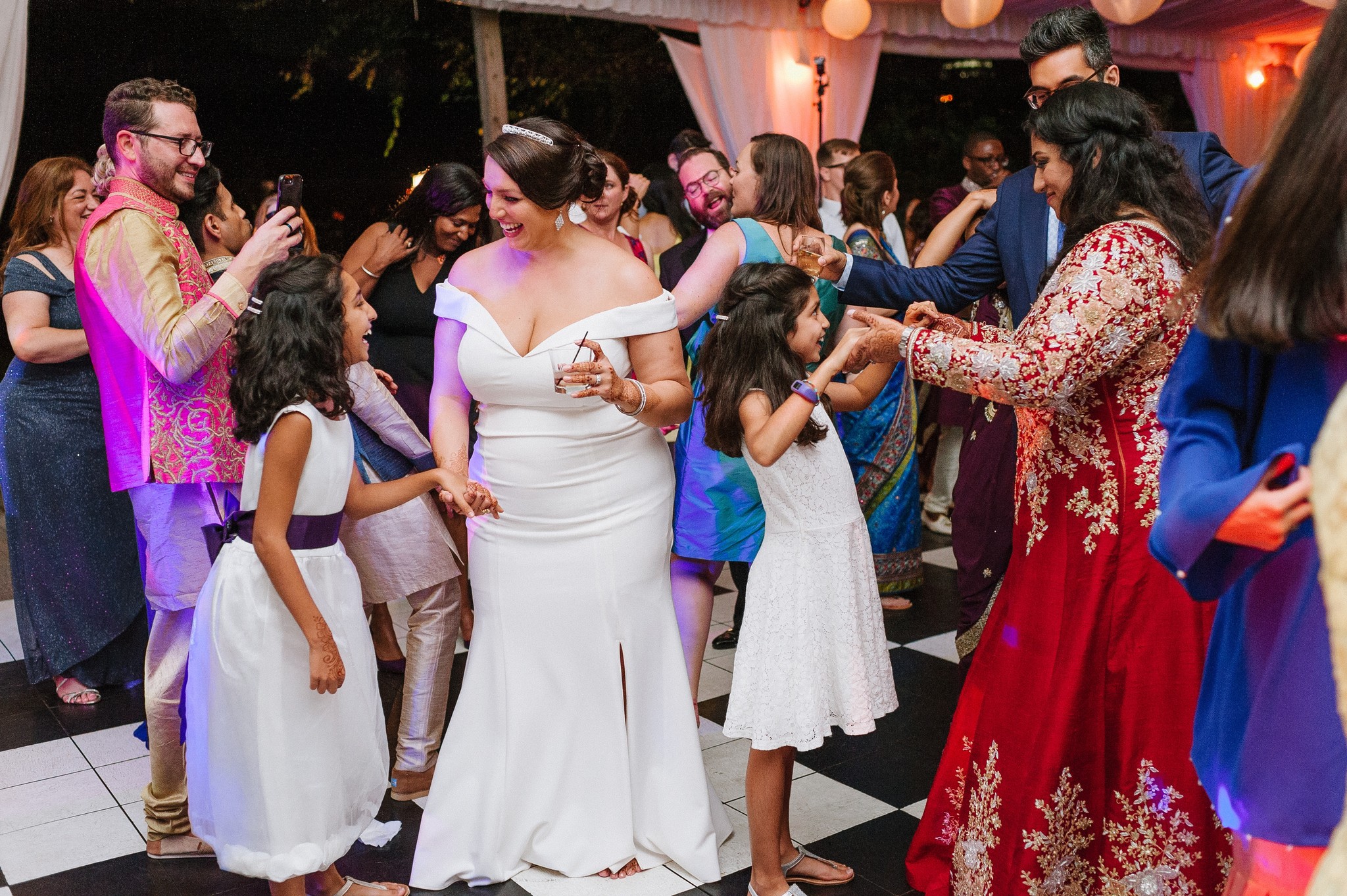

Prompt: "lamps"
[820,0,872,41]
[1090,1,1166,29]
[941,0,1006,30]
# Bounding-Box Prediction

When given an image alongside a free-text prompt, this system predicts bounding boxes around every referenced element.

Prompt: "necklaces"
[421,234,446,265]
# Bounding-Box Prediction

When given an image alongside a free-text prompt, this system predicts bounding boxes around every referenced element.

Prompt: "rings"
[593,374,601,386]
[479,506,491,515]
[284,222,293,233]
[405,238,413,248]
[869,359,877,365]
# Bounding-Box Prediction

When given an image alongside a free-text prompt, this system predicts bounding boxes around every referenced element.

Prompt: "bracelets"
[802,379,820,406]
[361,264,381,279]
[614,378,647,417]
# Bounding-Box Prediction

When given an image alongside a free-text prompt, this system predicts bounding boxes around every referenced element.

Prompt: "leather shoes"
[391,767,435,801]
[384,711,400,740]
[712,627,739,651]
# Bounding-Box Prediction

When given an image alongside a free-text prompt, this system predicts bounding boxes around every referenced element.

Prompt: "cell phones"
[273,173,306,249]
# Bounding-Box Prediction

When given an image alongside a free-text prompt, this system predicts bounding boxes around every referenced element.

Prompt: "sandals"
[748,881,808,896]
[775,844,855,887]
[304,876,411,896]
[53,674,101,705]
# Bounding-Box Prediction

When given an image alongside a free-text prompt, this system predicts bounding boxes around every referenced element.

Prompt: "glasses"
[126,130,214,158]
[970,156,1010,166]
[683,167,723,199]
[1024,65,1109,110]
[825,162,849,170]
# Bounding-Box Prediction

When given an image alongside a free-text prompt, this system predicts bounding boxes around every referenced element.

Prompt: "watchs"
[897,325,916,360]
[789,378,820,404]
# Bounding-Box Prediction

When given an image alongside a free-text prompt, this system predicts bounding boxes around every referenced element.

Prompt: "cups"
[797,236,825,276]
[548,347,592,394]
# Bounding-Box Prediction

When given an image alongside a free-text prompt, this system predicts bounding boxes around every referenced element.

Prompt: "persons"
[910,188,1015,680]
[836,76,1242,893]
[1,156,151,709]
[930,129,1021,240]
[73,75,304,862]
[174,163,469,804]
[545,126,712,289]
[839,150,928,610]
[669,134,871,729]
[185,252,505,894]
[1147,1,1346,896]
[703,264,944,896]
[424,115,705,881]
[805,138,912,270]
[250,194,322,262]
[657,147,745,372]
[328,161,482,460]
[786,8,1259,367]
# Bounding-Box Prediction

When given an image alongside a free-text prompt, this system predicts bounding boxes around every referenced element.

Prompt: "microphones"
[815,56,826,77]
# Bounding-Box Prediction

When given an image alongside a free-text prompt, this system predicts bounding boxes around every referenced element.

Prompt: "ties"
[1058,221,1068,255]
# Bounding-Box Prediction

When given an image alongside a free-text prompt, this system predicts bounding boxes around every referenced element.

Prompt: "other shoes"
[880,594,913,610]
[147,833,215,859]
[919,492,954,536]
[374,655,406,673]
